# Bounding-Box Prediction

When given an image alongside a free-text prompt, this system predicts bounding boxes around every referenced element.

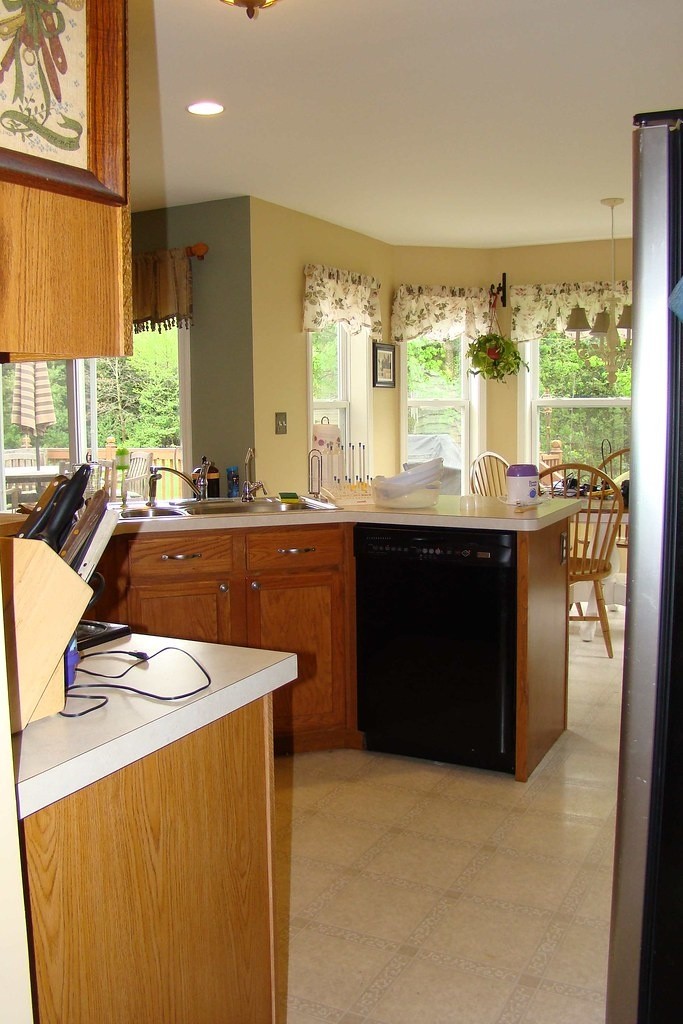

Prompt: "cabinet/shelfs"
[123,528,346,747]
[21,690,274,1024]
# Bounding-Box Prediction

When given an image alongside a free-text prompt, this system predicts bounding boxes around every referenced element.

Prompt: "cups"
[226,466,239,497]
[507,464,538,505]
[460,494,486,510]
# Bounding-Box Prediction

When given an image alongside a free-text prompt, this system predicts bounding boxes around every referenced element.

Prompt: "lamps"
[567,197,632,388]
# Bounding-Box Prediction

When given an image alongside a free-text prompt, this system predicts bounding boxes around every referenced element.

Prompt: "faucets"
[242,448,268,502]
[148,456,214,503]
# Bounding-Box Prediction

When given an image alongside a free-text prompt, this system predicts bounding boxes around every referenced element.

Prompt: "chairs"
[594,448,631,486]
[470,451,509,497]
[539,463,623,658]
[106,451,153,502]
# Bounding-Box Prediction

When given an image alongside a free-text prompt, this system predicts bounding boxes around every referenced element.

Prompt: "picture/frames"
[372,343,396,389]
[0,0,128,208]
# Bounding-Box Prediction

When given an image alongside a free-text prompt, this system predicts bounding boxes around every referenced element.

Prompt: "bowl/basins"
[370,480,440,510]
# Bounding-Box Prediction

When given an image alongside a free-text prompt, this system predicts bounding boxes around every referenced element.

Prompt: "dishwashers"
[349,522,518,780]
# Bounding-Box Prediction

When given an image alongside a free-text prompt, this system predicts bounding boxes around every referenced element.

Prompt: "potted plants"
[466,332,530,383]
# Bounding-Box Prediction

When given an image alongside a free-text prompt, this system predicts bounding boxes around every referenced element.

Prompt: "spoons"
[514,506,537,513]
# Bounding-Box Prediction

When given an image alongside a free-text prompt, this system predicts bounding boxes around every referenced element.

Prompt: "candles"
[114,440,132,470]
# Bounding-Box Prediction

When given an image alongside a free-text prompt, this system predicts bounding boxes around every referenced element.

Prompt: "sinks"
[187,500,344,517]
[123,508,184,518]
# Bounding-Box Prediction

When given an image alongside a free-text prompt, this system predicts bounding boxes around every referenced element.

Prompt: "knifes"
[14,464,119,583]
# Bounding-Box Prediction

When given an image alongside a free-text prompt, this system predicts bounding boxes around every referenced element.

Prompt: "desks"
[542,496,628,513]
[5,466,68,492]
